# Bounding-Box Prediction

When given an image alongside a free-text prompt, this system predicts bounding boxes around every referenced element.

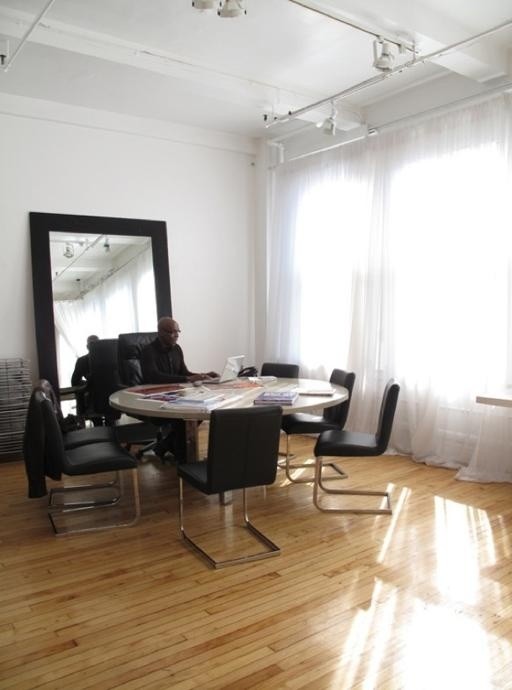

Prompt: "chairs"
[76,338,130,428]
[272,369,360,485]
[260,361,306,468]
[171,401,286,571]
[35,389,143,539]
[31,380,126,511]
[312,377,400,520]
[113,331,189,462]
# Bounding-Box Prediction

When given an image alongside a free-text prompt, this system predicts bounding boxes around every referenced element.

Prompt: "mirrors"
[28,209,180,435]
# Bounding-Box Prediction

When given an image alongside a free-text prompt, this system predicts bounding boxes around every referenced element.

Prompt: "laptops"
[195,355,245,384]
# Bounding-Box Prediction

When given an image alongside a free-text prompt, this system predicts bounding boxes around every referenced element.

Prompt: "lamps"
[189,0,405,74]
[315,98,341,138]
[63,240,76,257]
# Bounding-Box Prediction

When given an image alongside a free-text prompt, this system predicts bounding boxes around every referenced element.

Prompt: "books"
[160,387,223,411]
[299,388,336,396]
[253,391,298,405]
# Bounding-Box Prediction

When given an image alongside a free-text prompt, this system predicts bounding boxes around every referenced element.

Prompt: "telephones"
[237,366,257,378]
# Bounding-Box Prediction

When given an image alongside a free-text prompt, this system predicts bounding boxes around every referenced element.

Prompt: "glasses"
[160,327,182,335]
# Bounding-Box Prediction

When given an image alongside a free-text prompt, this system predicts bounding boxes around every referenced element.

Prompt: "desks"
[56,385,76,399]
[106,375,349,507]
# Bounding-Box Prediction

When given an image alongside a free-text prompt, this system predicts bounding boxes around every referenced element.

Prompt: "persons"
[69,334,112,427]
[140,318,219,465]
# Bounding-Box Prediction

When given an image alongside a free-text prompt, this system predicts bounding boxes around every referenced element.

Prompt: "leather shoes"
[150,441,188,463]
